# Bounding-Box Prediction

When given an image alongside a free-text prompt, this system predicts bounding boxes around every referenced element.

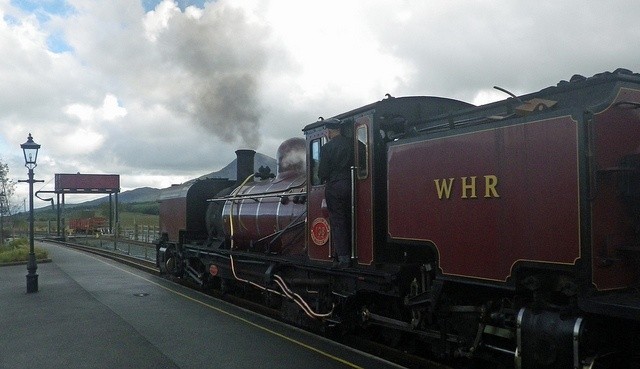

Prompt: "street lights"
[17,133,44,292]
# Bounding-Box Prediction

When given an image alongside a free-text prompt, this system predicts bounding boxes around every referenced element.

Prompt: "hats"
[323,118,343,129]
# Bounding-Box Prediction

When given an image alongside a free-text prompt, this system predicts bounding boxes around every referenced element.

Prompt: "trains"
[69,214,106,236]
[157,66,640,368]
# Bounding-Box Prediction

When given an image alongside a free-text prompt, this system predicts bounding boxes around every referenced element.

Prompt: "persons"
[318,119,365,267]
